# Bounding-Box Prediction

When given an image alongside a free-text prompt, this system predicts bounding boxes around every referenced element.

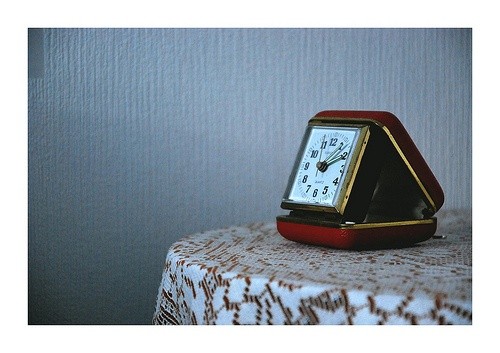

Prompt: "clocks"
[274,109,447,250]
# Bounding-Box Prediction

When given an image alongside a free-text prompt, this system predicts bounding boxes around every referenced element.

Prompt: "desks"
[152,210,472,324]
